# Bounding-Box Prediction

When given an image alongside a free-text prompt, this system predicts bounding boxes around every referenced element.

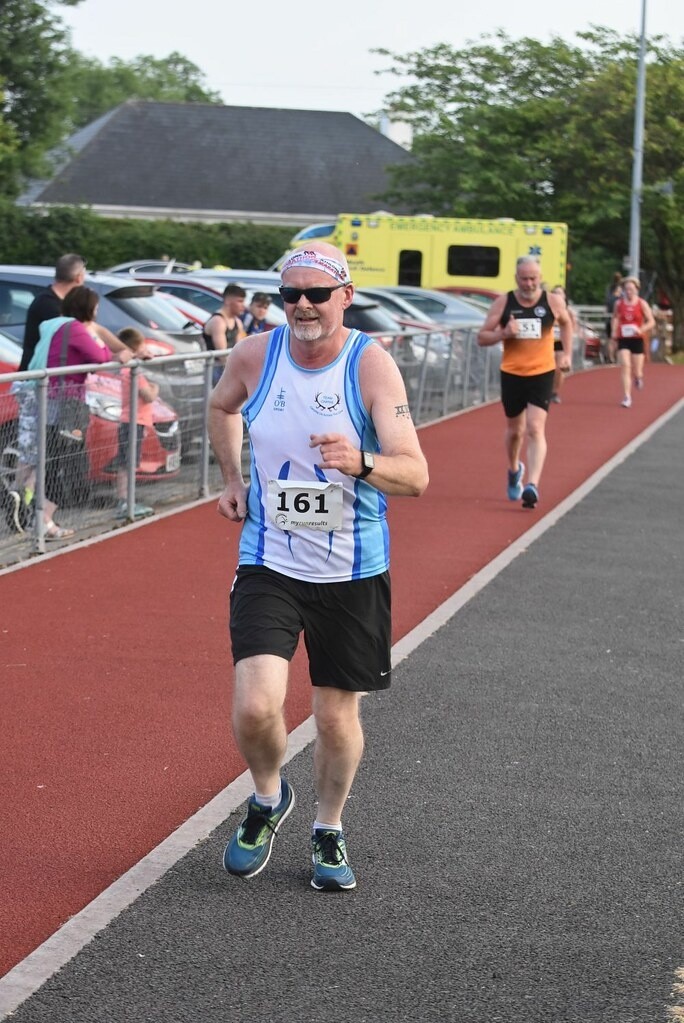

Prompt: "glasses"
[278,280,353,303]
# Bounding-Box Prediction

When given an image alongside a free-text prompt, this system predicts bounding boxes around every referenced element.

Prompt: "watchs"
[351,450,375,480]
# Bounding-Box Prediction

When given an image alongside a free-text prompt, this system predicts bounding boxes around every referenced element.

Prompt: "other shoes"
[6,490,28,533]
[553,394,561,404]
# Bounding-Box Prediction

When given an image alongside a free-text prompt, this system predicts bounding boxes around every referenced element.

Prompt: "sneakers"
[621,396,632,407]
[310,828,356,889]
[506,460,525,500]
[115,500,153,519]
[521,484,539,508]
[222,778,295,878]
[635,377,643,390]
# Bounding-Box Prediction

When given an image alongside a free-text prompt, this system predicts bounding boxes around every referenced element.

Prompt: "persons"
[551,273,664,409]
[476,256,573,509]
[207,243,431,892]
[5,253,270,542]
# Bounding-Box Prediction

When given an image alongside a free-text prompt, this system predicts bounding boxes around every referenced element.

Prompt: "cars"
[95,261,601,443]
[0,328,181,510]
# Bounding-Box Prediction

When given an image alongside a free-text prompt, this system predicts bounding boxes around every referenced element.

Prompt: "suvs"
[0,263,210,468]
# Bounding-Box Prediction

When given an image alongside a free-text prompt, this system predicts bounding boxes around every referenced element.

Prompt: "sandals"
[32,519,76,541]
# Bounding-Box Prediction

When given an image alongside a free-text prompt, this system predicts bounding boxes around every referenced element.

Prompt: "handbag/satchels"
[53,399,92,442]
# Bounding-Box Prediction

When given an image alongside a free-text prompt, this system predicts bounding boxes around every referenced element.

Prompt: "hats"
[254,293,273,302]
